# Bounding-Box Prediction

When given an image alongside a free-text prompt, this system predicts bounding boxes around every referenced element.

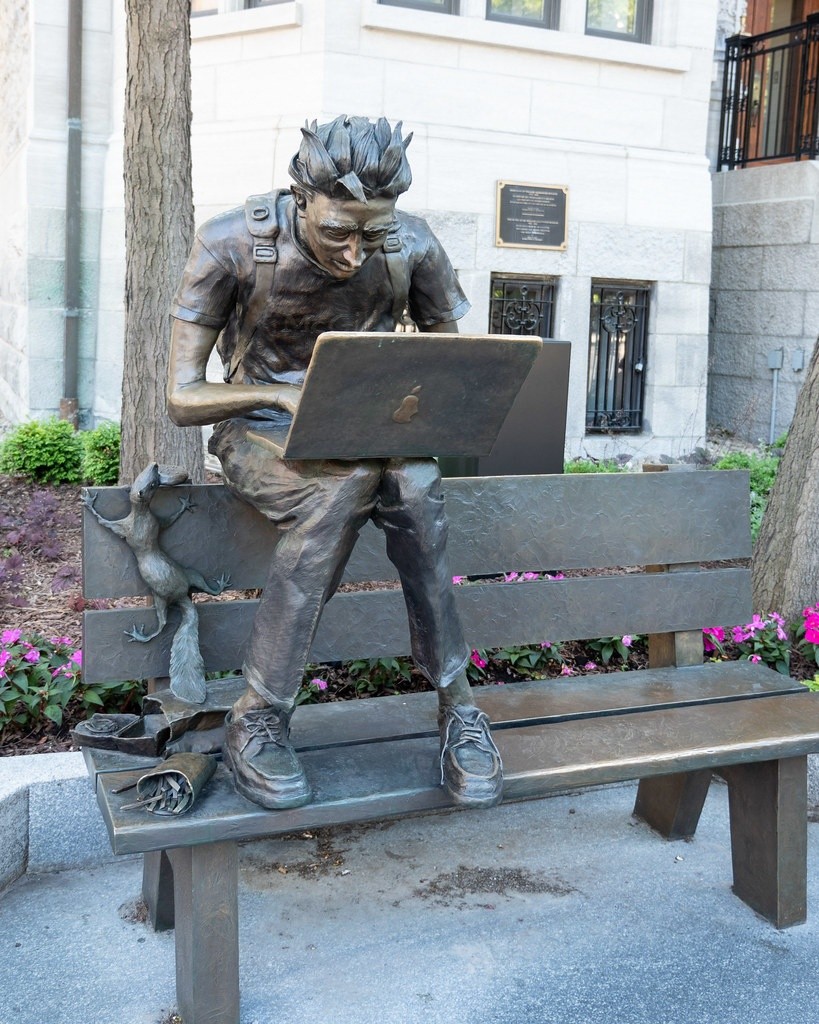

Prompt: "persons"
[162,112,504,810]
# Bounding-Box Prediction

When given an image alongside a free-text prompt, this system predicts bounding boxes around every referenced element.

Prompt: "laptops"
[247,331,542,460]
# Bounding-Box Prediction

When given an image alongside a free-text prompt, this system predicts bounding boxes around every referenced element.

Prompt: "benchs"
[78,466,818,1023]
